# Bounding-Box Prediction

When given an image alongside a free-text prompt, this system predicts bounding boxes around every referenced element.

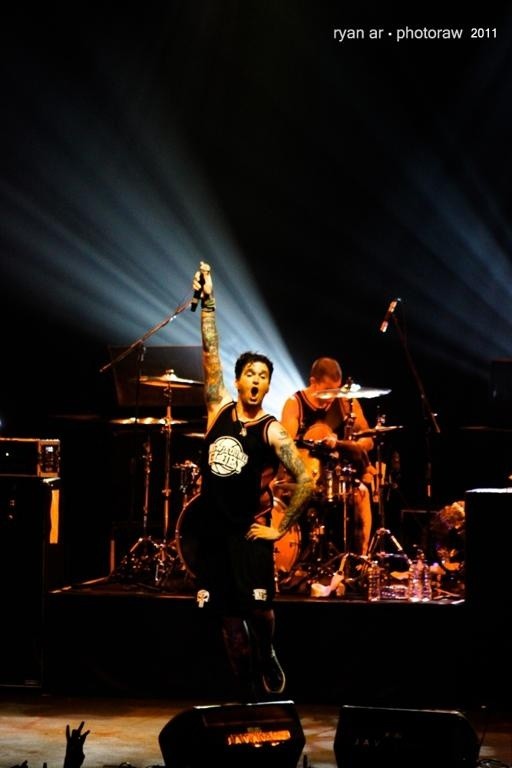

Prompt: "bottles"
[366,553,432,602]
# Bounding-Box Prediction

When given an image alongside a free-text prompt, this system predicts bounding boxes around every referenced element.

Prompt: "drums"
[183,433,203,471]
[176,492,300,580]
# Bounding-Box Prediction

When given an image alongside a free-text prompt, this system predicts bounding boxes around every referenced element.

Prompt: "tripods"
[167,460,202,541]
[109,438,181,586]
[127,382,194,597]
[295,400,460,598]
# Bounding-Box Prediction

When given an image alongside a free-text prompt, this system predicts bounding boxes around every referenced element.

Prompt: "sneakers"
[255,646,286,694]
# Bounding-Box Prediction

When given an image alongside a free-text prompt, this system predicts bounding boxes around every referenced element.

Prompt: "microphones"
[378,302,401,333]
[190,263,210,315]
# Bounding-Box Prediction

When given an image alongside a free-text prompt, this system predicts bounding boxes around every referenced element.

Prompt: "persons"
[12,721,91,768]
[281,358,375,595]
[192,260,314,703]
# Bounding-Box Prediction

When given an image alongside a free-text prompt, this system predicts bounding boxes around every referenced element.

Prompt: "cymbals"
[352,427,402,438]
[142,373,204,389]
[115,417,187,425]
[314,384,390,399]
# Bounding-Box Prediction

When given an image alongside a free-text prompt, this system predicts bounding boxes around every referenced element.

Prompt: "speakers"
[156,702,309,767]
[333,705,479,768]
